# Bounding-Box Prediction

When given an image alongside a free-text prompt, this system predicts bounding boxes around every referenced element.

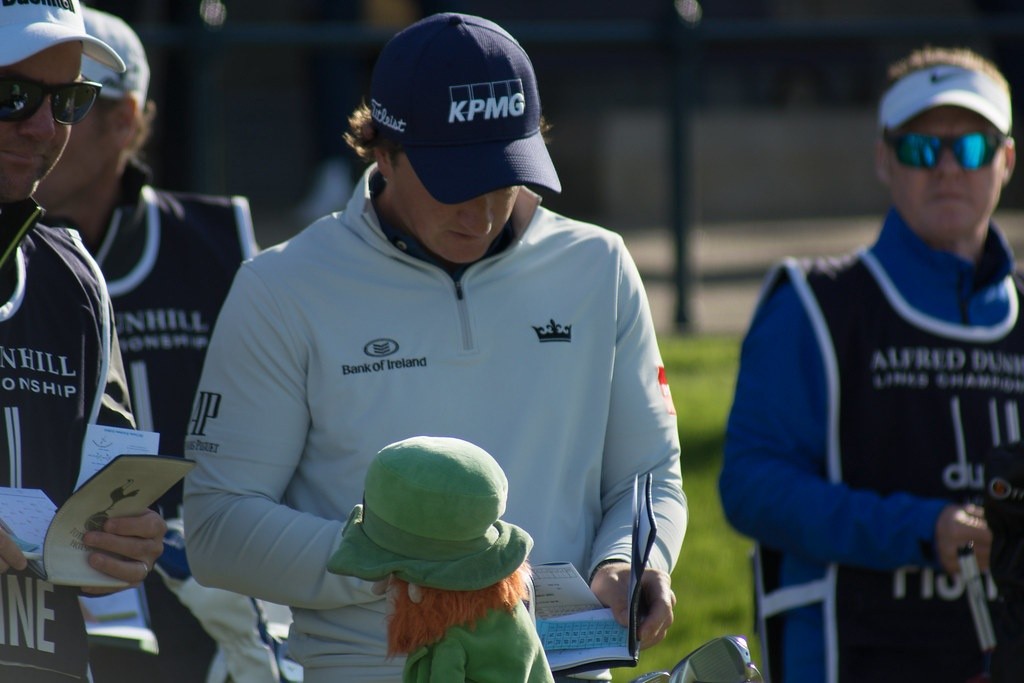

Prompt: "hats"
[327,436,534,592]
[1,1,131,75]
[874,63,1014,137]
[75,3,150,112]
[368,7,563,205]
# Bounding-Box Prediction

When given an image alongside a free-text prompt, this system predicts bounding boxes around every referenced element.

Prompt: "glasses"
[0,69,105,126]
[881,128,1006,175]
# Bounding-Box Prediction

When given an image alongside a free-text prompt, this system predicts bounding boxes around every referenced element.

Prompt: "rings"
[142,562,148,577]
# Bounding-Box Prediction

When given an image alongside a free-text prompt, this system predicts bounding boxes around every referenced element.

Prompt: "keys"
[955,540,981,583]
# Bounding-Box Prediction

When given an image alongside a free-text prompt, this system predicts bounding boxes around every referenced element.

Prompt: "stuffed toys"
[327,435,555,683]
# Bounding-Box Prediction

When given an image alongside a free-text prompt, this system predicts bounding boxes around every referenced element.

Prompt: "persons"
[184,11,689,683]
[0,0,305,683]
[717,45,1024,683]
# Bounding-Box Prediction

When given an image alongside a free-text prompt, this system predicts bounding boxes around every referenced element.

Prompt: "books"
[0,423,197,588]
[536,472,657,676]
